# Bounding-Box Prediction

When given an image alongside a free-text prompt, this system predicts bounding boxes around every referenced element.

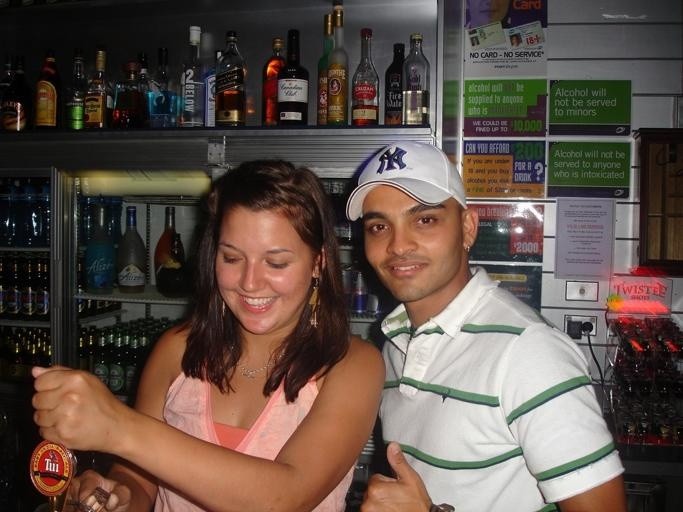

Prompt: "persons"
[345,142,626,512]
[33,159,386,511]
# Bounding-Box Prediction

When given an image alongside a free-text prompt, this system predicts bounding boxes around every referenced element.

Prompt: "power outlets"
[563,314,597,336]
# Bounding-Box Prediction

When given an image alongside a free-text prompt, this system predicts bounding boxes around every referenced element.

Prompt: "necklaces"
[227,335,286,379]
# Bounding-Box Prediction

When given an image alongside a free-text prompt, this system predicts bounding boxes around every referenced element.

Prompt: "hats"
[344,138,467,221]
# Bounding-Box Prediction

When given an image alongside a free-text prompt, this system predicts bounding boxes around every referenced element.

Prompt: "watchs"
[429,503,455,512]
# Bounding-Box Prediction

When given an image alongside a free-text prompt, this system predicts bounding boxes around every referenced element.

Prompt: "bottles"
[7,261,22,316]
[82,206,115,295]
[384,43,406,125]
[22,262,37,320]
[262,36,286,128]
[339,263,354,306]
[155,47,171,92]
[201,49,224,128]
[115,206,148,294]
[350,27,381,127]
[176,25,204,128]
[402,33,430,128]
[114,49,153,129]
[153,206,186,298]
[351,269,370,314]
[37,264,50,321]
[77,257,87,314]
[0,262,7,319]
[38,184,51,247]
[326,0,350,127]
[32,53,63,131]
[84,49,114,130]
[21,184,43,248]
[0,186,21,248]
[0,326,53,389]
[62,56,89,130]
[276,28,310,124]
[315,12,335,128]
[214,31,248,127]
[78,314,175,397]
[35,263,43,289]
[0,61,30,134]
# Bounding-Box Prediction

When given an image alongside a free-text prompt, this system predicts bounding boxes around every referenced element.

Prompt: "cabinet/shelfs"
[0,126,57,512]
[57,127,437,485]
